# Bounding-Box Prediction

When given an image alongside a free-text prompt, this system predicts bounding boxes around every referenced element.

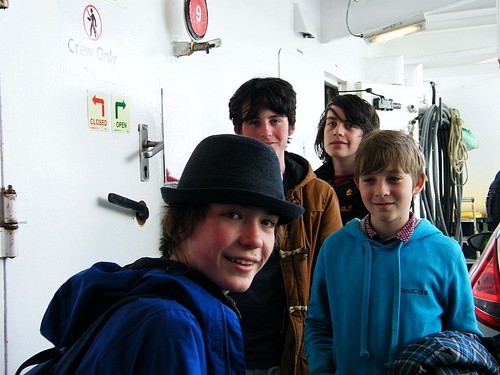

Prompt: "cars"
[466,221,500,368]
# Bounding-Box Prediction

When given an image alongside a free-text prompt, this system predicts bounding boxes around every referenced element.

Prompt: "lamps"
[362,16,428,46]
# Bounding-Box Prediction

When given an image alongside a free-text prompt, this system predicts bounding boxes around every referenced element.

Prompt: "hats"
[159,133,304,224]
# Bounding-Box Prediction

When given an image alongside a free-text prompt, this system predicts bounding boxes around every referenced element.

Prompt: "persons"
[312,94,380,224]
[305,130,486,375]
[41,134,304,375]
[226,77,344,375]
